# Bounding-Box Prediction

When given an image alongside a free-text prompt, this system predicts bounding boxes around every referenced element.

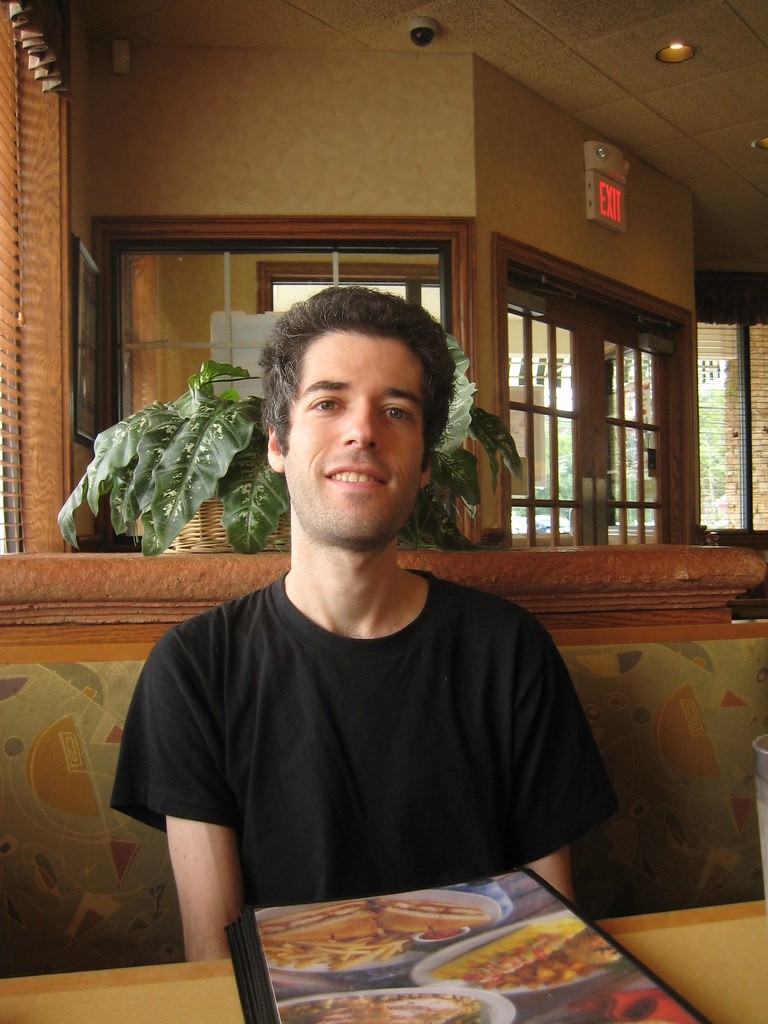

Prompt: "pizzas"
[276,994,484,1024]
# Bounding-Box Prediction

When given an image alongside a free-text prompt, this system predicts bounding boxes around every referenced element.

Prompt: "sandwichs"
[378,900,490,933]
[258,898,380,942]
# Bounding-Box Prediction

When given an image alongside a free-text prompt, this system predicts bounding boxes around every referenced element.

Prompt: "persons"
[111,285,616,963]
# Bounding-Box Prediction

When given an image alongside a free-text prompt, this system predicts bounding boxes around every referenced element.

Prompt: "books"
[225,868,712,1024]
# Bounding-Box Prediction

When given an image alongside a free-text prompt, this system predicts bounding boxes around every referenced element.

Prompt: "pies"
[428,919,586,982]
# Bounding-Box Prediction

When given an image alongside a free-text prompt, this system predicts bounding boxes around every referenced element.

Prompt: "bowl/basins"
[414,924,469,944]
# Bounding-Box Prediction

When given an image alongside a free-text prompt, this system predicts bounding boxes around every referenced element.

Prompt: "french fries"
[263,927,413,970]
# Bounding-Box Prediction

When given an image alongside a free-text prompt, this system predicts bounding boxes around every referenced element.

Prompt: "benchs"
[1,545,768,981]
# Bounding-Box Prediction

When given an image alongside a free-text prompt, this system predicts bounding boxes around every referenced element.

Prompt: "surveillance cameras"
[408,17,437,47]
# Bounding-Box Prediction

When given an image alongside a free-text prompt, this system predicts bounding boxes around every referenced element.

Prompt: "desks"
[0,900,768,1024]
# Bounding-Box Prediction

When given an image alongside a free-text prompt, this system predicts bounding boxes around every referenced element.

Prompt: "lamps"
[583,139,630,186]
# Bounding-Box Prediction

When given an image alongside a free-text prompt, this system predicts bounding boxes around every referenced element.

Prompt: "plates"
[409,909,624,995]
[254,889,502,972]
[277,987,516,1024]
